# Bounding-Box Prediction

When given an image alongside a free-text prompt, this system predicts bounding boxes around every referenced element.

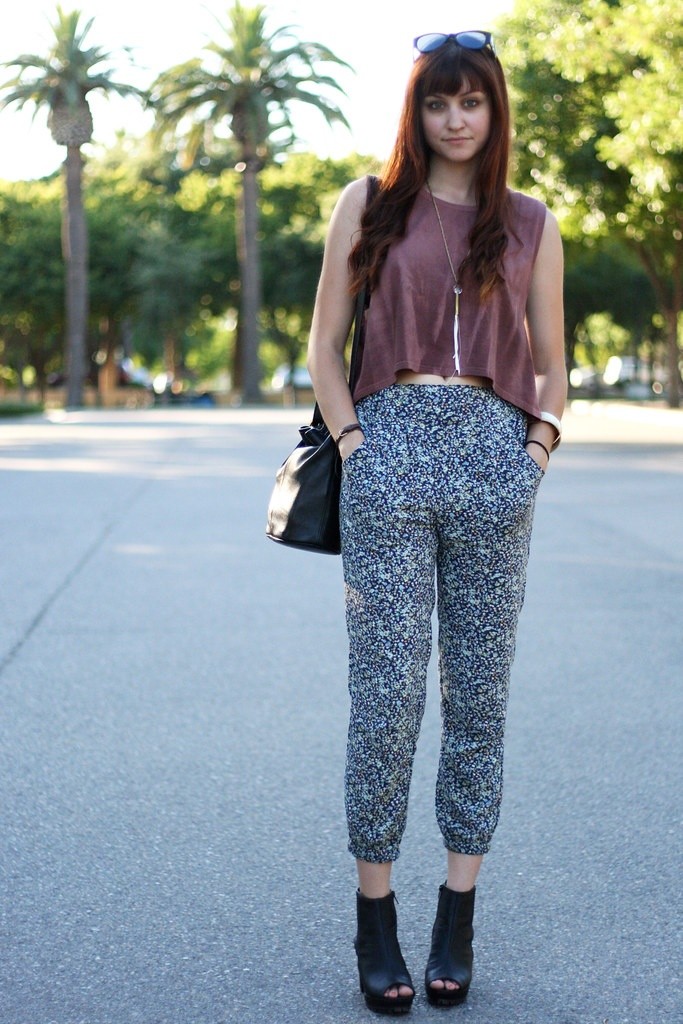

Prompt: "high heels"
[424,881,475,1005]
[353,887,415,1015]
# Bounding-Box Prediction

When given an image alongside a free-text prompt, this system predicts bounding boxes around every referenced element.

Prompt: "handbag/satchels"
[267,400,340,556]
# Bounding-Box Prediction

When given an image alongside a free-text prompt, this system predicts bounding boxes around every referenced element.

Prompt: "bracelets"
[526,440,549,461]
[538,410,562,454]
[332,423,360,446]
[422,174,478,379]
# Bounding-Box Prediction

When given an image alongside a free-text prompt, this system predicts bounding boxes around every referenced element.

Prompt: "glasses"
[412,31,494,54]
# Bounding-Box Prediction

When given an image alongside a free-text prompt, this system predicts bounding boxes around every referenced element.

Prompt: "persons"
[301,33,568,1015]
